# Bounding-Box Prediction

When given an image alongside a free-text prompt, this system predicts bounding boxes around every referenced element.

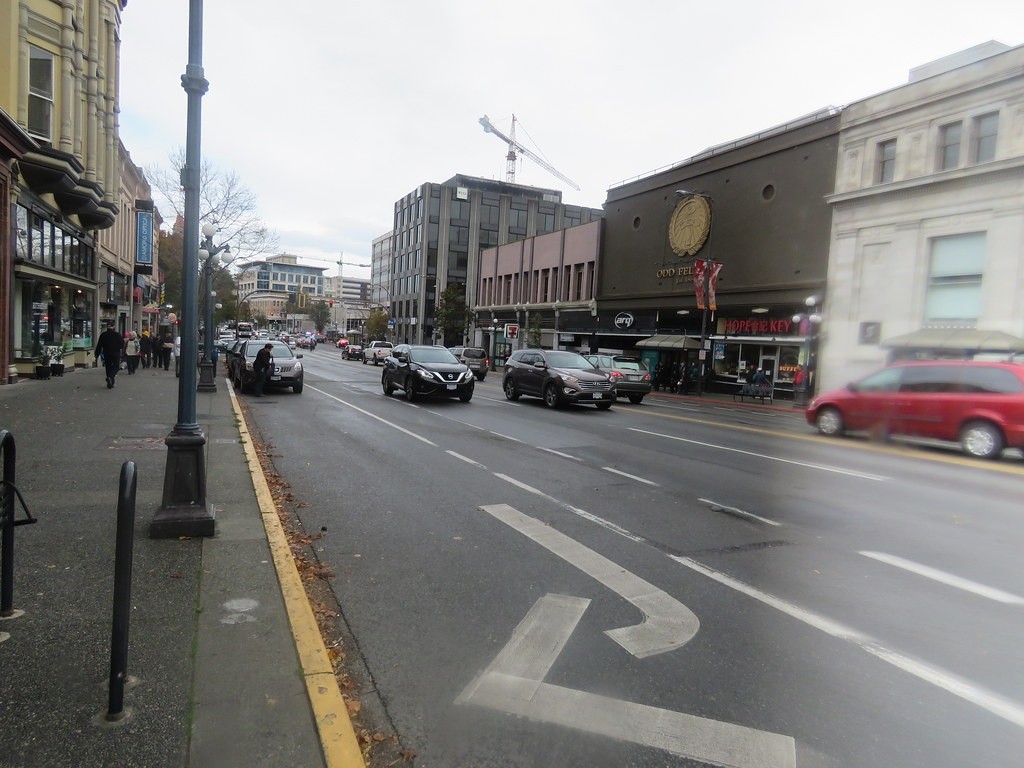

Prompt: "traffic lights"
[298,294,305,308]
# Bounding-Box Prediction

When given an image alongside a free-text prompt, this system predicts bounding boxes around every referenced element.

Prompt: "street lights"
[790,296,822,410]
[677,188,716,396]
[489,318,501,371]
[199,226,232,391]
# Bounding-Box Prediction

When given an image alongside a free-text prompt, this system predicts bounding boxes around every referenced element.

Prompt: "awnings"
[636,335,700,351]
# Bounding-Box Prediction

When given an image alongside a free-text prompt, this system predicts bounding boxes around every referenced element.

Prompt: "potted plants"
[51,342,72,377]
[36,346,56,380]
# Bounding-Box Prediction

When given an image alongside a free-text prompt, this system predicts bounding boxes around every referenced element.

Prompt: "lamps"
[15,228,27,235]
[50,212,64,224]
[75,230,86,238]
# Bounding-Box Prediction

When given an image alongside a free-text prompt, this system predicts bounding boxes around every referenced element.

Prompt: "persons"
[122,331,181,377]
[253,344,274,397]
[746,365,766,392]
[652,361,716,394]
[93,322,125,389]
[793,364,813,396]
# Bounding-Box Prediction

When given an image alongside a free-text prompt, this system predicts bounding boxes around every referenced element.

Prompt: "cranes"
[233,245,371,299]
[479,113,583,191]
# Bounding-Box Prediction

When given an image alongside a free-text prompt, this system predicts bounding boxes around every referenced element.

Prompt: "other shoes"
[256,394,267,396]
[106,376,112,389]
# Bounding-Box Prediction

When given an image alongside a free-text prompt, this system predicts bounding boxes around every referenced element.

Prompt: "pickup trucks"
[362,341,394,366]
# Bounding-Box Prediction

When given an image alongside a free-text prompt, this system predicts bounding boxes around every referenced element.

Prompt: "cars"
[255,329,326,349]
[341,345,364,360]
[336,339,350,348]
[197,329,237,350]
[30,320,92,338]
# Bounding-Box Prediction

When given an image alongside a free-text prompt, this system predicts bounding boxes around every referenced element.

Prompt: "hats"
[143,331,149,336]
[107,323,115,327]
[757,368,762,370]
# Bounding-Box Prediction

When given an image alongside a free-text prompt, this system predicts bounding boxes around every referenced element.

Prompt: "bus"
[238,322,252,338]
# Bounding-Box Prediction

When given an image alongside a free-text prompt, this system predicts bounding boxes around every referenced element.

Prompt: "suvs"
[381,344,474,403]
[806,359,1024,458]
[446,346,489,382]
[226,339,305,394]
[501,348,616,410]
[581,353,652,403]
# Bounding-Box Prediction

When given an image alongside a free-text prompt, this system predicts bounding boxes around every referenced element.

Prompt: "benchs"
[733,382,774,404]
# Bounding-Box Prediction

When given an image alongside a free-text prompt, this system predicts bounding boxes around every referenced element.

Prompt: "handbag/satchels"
[266,356,275,376]
